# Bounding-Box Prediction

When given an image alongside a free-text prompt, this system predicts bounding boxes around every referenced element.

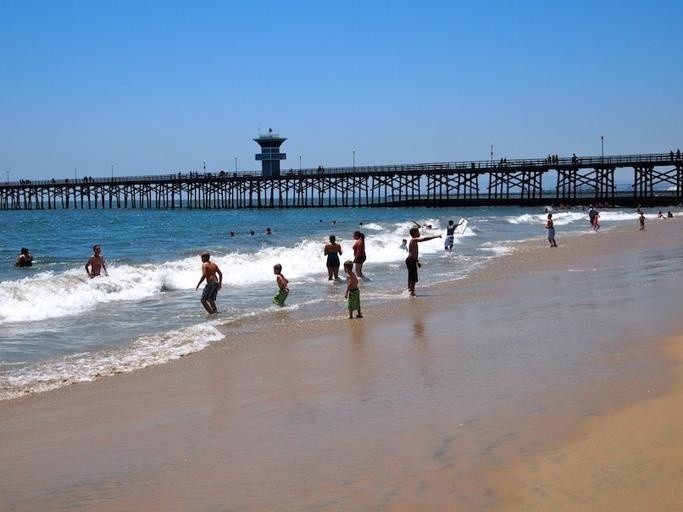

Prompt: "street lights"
[298,156,302,171]
[234,155,239,175]
[352,150,356,171]
[111,163,115,177]
[203,160,206,175]
[601,136,604,158]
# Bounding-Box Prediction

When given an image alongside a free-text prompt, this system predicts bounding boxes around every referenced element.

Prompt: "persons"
[315,214,466,320]
[540,199,673,231]
[670,150,675,161]
[572,154,578,165]
[545,212,560,249]
[195,252,223,315]
[85,244,110,279]
[15,247,34,268]
[272,264,289,309]
[547,153,559,165]
[676,149,681,161]
[225,227,272,235]
